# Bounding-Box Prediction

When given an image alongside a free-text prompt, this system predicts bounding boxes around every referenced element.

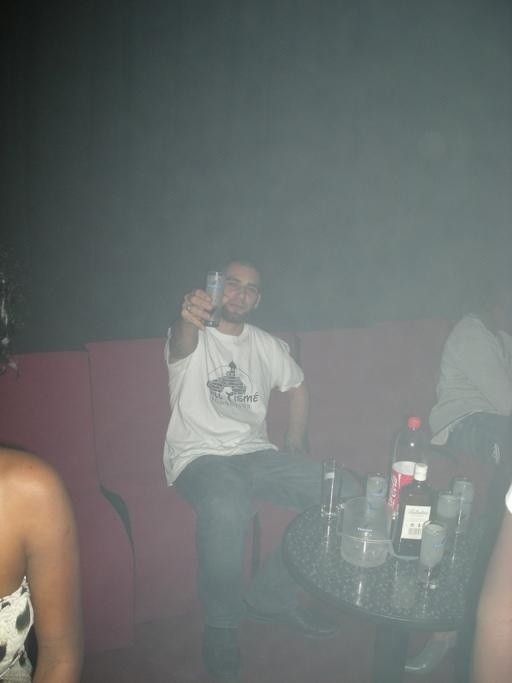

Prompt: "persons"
[164,258,337,677]
[464,484,512,682]
[1,271,88,682]
[429,266,511,579]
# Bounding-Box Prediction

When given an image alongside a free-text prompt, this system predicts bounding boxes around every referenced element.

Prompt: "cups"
[451,476,476,538]
[318,458,345,521]
[201,269,226,328]
[433,489,463,553]
[363,471,390,519]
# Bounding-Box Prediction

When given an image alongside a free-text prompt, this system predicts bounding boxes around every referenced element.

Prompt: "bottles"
[391,462,434,557]
[386,416,430,530]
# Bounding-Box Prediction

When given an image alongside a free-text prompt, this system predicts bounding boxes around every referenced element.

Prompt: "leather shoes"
[243,594,339,636]
[404,638,457,674]
[202,624,241,682]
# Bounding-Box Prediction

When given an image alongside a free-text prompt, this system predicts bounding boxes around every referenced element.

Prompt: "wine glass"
[416,519,448,585]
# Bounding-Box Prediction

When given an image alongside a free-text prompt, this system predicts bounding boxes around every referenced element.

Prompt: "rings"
[186,304,192,311]
